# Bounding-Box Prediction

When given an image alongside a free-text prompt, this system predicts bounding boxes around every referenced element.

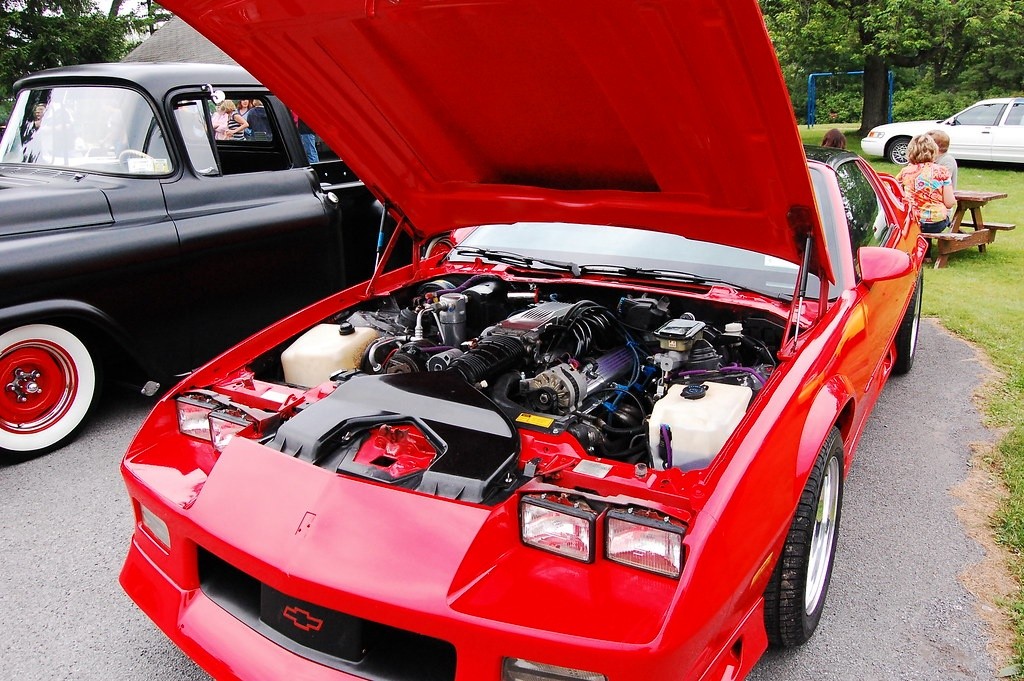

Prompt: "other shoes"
[923,258,932,263]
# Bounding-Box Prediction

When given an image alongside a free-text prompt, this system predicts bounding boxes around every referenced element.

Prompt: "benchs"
[920,231,979,240]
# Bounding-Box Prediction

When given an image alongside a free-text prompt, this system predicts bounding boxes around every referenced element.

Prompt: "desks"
[961,219,1015,230]
[933,189,1008,269]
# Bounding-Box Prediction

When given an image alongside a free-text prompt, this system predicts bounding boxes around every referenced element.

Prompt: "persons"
[821,128,846,149]
[212,99,319,164]
[30,103,46,139]
[895,130,957,233]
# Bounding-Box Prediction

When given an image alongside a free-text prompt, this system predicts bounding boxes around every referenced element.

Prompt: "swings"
[828,75,840,120]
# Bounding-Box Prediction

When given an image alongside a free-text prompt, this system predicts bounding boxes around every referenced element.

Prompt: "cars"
[861,97,1023,172]
[0,61,413,458]
[114,0,932,681]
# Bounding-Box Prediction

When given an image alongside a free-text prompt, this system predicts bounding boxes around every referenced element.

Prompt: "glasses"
[34,103,46,108]
